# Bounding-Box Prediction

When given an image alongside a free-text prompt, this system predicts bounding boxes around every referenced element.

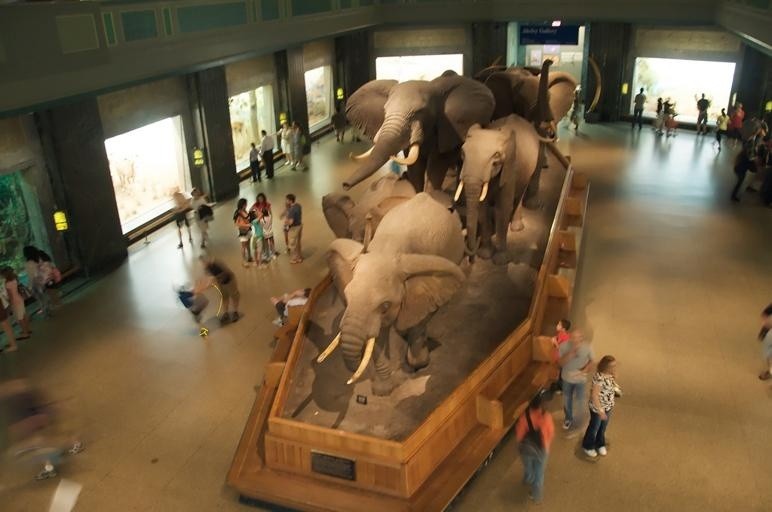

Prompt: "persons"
[633,87,645,129]
[0,246,61,354]
[582,354,623,457]
[759,302,772,381]
[516,394,555,500]
[656,93,772,203]
[331,107,345,142]
[552,318,571,394]
[171,120,311,337]
[558,332,595,429]
[573,99,585,129]
[351,126,362,142]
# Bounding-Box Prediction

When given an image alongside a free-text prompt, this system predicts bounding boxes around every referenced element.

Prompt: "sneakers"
[598,446,607,456]
[583,448,598,457]
[563,420,572,429]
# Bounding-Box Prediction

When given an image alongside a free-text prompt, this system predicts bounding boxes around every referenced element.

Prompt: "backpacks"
[519,407,545,464]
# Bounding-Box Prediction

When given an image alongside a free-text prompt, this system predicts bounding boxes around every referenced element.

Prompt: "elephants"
[450,111,541,269]
[320,173,418,243]
[340,68,498,199]
[314,189,484,390]
[475,58,578,211]
